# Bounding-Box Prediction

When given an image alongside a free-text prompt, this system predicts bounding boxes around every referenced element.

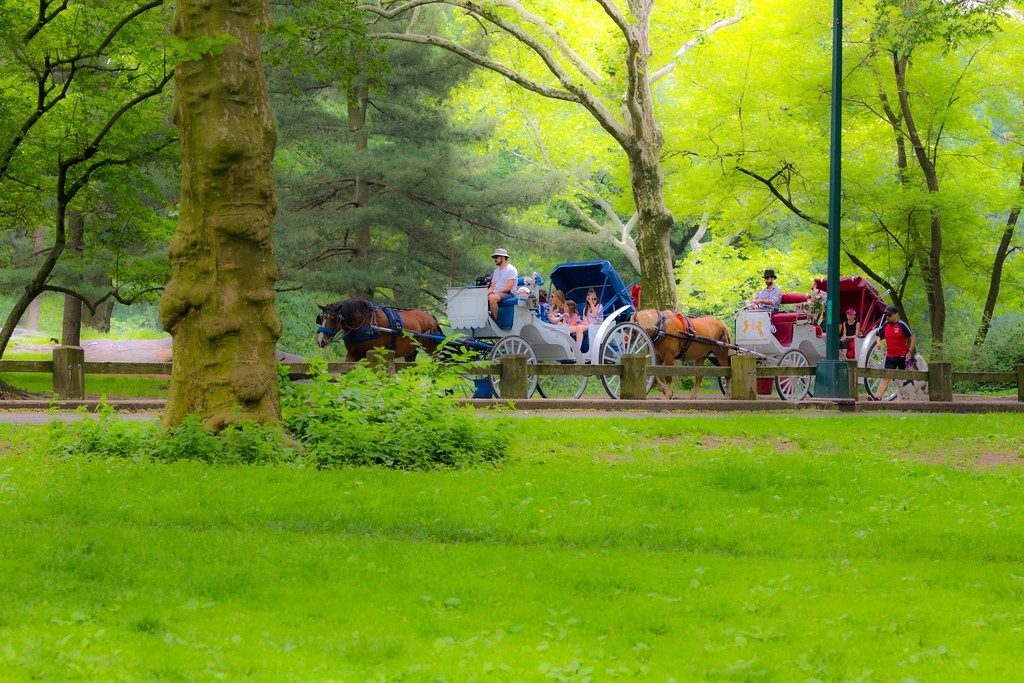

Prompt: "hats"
[491,248,510,258]
[883,307,899,314]
[762,270,777,279]
[846,309,856,315]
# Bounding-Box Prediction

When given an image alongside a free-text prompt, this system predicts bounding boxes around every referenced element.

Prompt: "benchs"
[570,301,615,352]
[770,292,811,346]
[846,320,874,358]
[497,276,533,329]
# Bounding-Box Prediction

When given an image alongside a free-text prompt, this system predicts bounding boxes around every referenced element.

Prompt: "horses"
[624,308,735,400]
[315,297,447,374]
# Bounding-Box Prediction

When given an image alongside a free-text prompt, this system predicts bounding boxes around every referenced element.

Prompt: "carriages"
[313,258,657,400]
[620,276,913,400]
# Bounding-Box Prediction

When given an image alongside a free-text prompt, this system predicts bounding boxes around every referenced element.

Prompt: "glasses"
[551,295,556,298]
[885,313,892,317]
[594,298,597,300]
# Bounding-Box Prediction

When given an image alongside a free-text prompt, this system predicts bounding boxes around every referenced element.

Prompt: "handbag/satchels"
[839,340,848,361]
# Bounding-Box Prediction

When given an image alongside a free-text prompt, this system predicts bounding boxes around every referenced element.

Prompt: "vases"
[757,377,773,394]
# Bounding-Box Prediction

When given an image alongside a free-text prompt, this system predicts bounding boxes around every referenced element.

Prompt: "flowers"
[805,286,827,317]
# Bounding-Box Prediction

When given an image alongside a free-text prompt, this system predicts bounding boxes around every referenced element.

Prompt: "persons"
[747,269,782,332]
[549,290,567,316]
[558,301,580,333]
[868,306,914,401]
[539,290,569,328]
[487,248,518,319]
[576,288,602,350]
[819,311,843,349]
[519,287,530,298]
[900,346,929,400]
[840,309,860,357]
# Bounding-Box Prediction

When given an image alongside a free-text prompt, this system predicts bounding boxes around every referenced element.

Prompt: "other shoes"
[868,395,879,401]
